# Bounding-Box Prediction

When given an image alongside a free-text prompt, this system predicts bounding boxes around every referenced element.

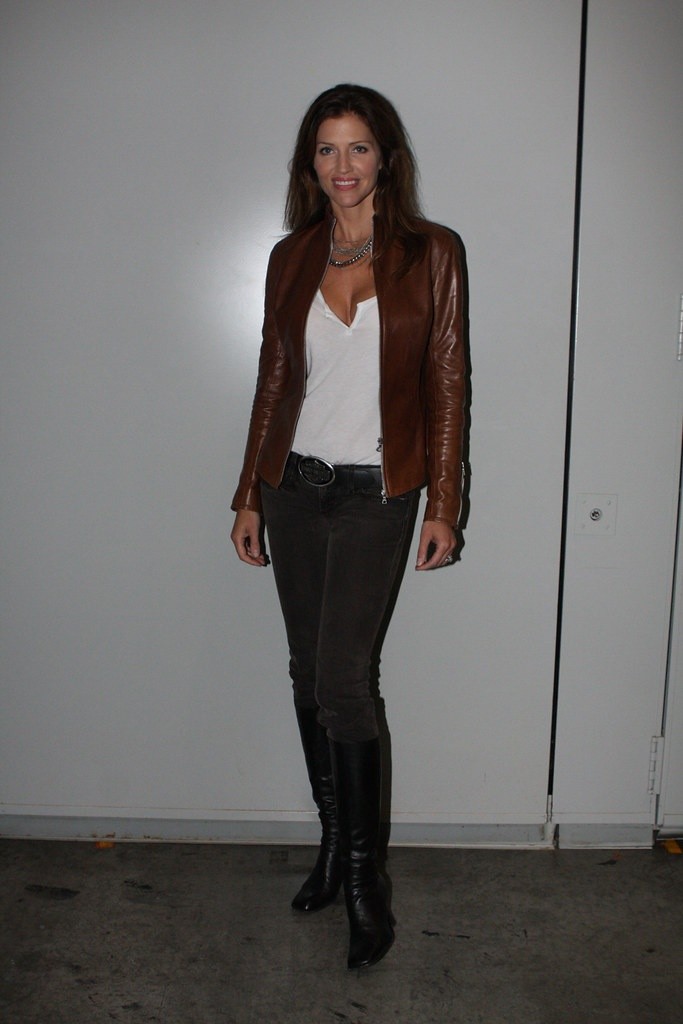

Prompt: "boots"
[328,733,396,970]
[290,738,343,911]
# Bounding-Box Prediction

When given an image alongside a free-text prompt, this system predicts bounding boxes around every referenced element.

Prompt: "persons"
[229,81,475,973]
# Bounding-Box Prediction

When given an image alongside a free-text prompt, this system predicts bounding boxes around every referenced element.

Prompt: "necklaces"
[328,234,375,270]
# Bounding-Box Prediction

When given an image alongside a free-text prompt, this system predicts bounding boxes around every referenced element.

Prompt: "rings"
[443,554,453,563]
